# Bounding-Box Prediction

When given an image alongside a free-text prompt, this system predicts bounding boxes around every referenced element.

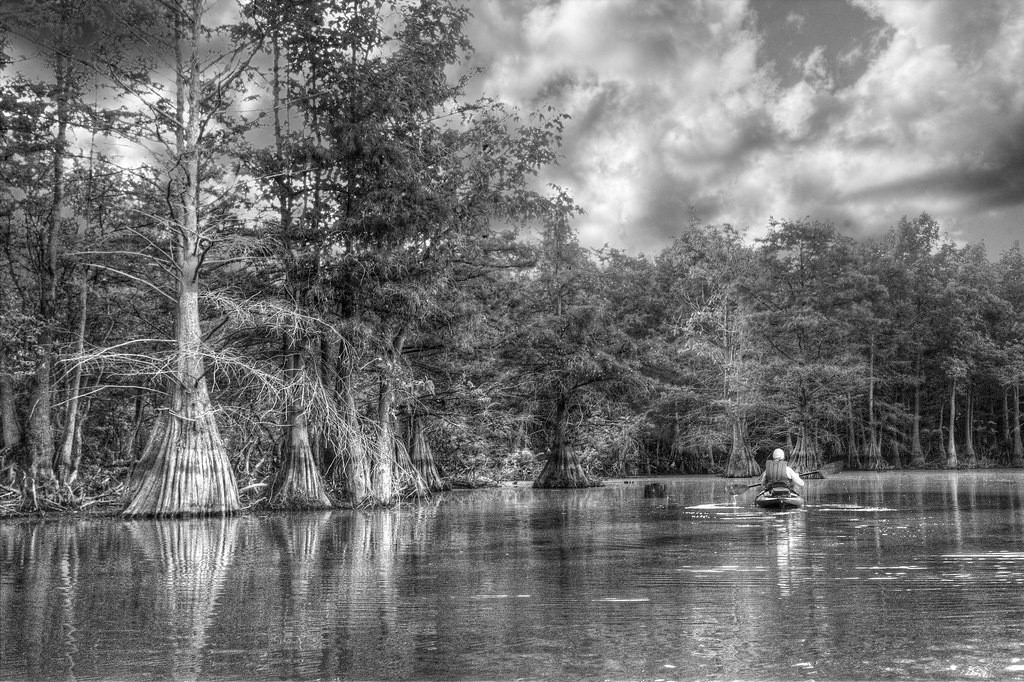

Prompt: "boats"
[756,484,806,510]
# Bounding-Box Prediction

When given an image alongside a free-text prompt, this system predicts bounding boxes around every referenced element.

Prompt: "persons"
[759,447,806,495]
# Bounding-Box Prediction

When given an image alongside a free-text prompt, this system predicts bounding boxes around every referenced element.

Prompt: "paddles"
[725,470,826,496]
